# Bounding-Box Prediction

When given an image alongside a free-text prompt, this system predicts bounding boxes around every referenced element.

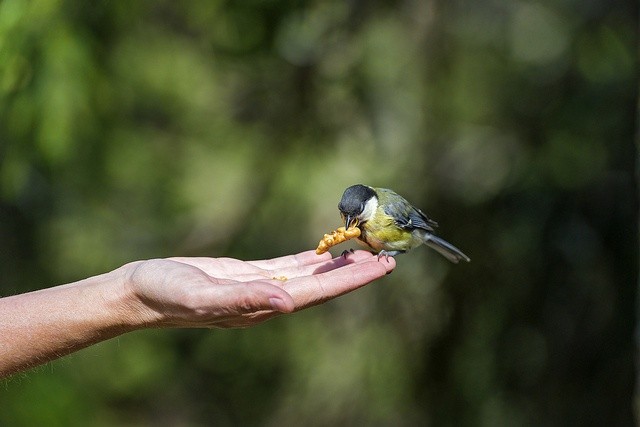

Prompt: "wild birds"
[337,184,471,265]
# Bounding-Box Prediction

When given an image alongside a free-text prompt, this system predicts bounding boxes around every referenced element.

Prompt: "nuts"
[315,226,361,255]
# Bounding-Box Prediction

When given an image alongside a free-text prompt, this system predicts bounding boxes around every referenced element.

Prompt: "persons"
[0,250,396,380]
